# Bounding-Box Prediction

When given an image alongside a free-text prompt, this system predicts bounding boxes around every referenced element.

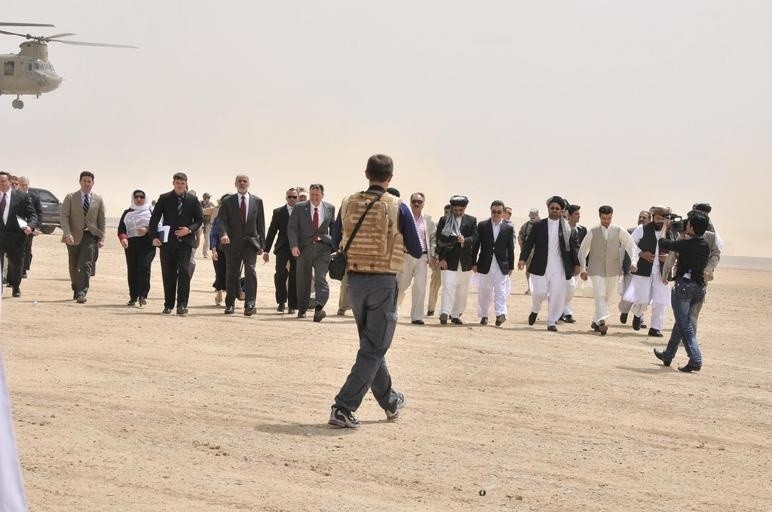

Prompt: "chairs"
[328,404,362,428]
[385,393,406,420]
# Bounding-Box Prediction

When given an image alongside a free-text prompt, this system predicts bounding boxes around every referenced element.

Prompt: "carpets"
[0,193,6,216]
[177,197,183,242]
[82,194,89,228]
[313,208,318,241]
[240,196,245,223]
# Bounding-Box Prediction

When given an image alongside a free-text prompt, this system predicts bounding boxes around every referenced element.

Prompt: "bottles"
[529,208,538,218]
[296,186,308,196]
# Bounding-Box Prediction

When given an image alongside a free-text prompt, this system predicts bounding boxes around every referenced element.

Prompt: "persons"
[117,172,517,327]
[0,173,43,301]
[58,169,106,304]
[517,195,719,341]
[328,156,422,426]
[653,210,710,373]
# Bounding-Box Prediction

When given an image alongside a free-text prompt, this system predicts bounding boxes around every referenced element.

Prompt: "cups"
[492,211,502,213]
[412,200,422,204]
[288,195,297,199]
[550,207,560,210]
[134,196,144,199]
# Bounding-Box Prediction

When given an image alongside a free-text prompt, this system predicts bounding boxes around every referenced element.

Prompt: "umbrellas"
[678,364,701,372]
[592,319,608,335]
[496,315,505,326]
[412,311,462,325]
[654,348,672,366]
[13,288,20,297]
[529,312,576,331]
[620,313,663,336]
[215,290,256,316]
[481,317,488,324]
[73,292,87,302]
[277,303,325,322]
[163,305,188,314]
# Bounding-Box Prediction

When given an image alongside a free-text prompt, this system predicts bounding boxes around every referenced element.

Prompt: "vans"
[128,296,146,307]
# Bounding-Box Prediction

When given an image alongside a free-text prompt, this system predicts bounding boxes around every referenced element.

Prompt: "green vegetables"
[0,22,140,110]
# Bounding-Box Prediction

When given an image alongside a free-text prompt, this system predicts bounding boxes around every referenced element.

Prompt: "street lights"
[329,251,346,280]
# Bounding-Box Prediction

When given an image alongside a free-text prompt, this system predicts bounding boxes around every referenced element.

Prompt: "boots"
[27,188,63,235]
